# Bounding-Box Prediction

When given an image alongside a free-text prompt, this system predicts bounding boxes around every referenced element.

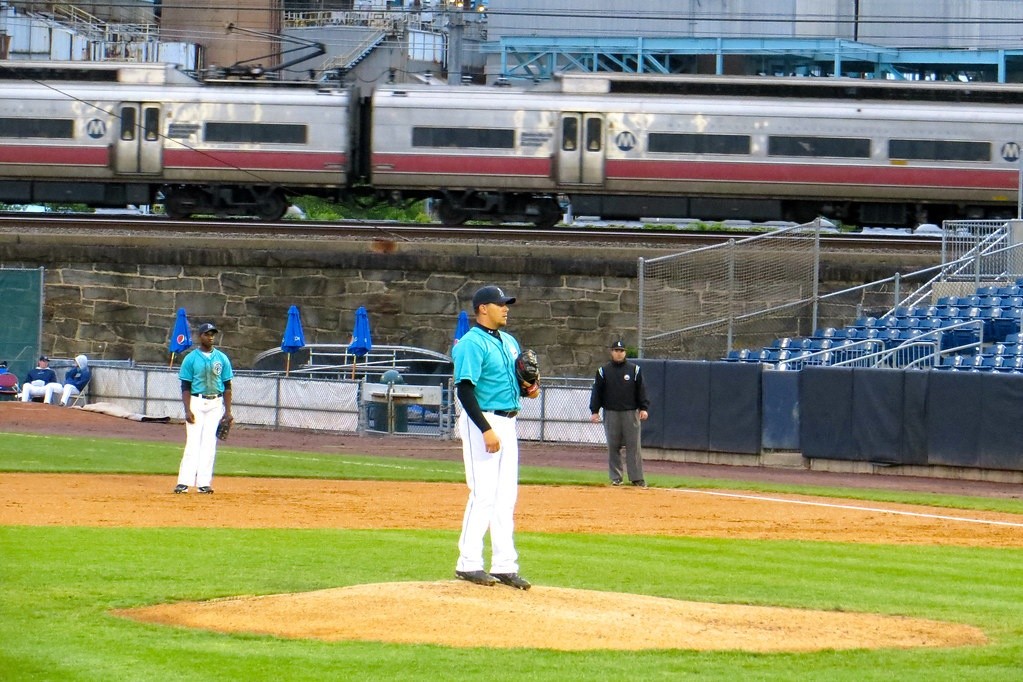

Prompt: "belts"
[482,410,518,419]
[192,393,223,400]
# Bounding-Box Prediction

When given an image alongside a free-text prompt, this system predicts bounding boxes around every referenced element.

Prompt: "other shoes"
[174,485,189,493]
[198,486,214,494]
[454,569,498,587]
[633,480,647,488]
[59,402,65,406]
[489,573,532,591]
[611,478,623,486]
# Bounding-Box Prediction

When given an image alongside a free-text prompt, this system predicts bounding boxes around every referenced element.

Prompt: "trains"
[0,78,1023,229]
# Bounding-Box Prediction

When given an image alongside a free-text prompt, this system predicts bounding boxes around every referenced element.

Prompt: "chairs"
[0,372,95,407]
[721,277,1023,374]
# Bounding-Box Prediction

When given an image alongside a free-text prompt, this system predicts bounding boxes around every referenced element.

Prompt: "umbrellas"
[168,308,192,371]
[453,310,470,345]
[281,306,305,378]
[348,306,370,380]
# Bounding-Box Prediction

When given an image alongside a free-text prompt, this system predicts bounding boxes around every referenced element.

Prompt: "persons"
[452,286,539,590]
[174,323,233,494]
[22,355,56,403]
[44,354,89,407]
[589,340,648,487]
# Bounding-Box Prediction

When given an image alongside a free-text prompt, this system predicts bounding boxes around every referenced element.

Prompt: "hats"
[611,341,628,351]
[199,324,218,335]
[473,286,517,309]
[40,356,50,362]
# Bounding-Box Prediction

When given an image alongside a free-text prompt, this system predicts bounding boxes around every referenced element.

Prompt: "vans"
[252,343,464,426]
